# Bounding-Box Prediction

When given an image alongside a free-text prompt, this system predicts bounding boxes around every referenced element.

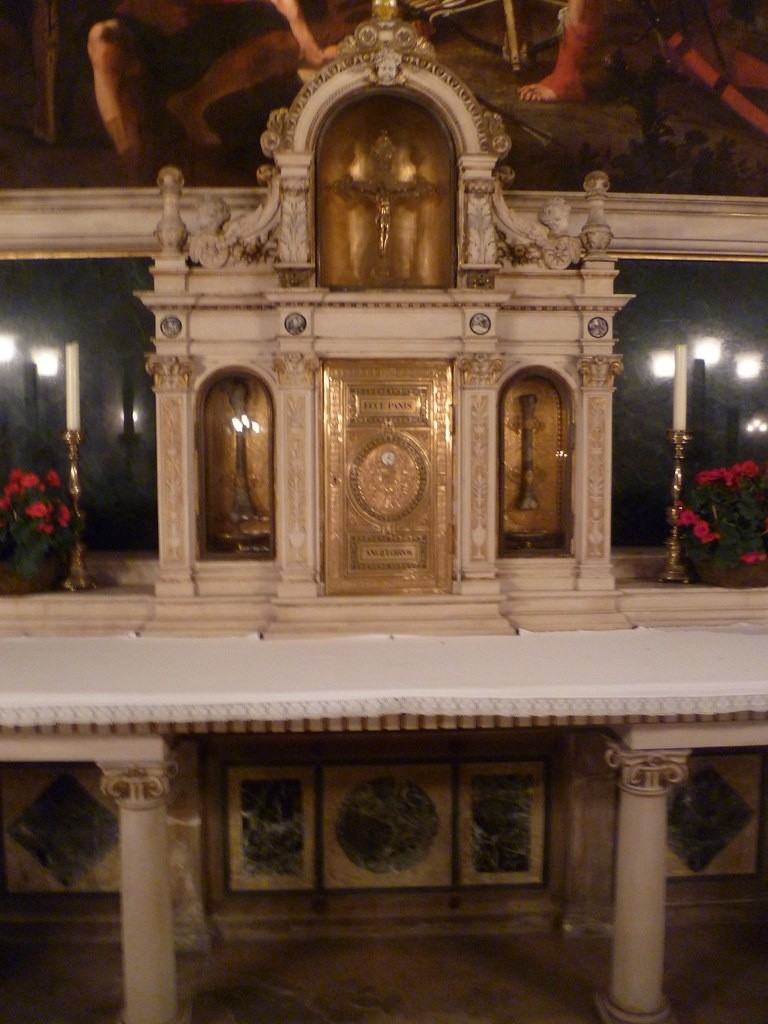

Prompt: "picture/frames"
[0,759,120,900]
[221,759,320,896]
[662,750,767,883]
[454,756,551,886]
[316,757,456,894]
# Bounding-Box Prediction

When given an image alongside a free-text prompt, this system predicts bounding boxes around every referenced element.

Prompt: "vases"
[702,561,768,590]
[0,560,56,596]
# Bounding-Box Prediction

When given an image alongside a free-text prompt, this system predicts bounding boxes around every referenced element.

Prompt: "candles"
[65,340,80,431]
[672,343,688,430]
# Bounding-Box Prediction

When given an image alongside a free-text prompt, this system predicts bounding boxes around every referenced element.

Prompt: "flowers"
[0,467,79,578]
[674,459,768,585]
